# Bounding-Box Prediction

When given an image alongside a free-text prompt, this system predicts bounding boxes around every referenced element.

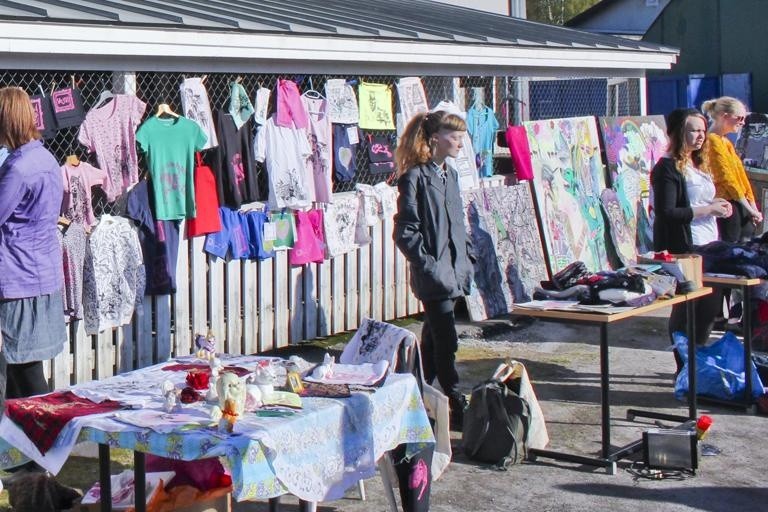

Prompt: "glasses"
[728,113,746,123]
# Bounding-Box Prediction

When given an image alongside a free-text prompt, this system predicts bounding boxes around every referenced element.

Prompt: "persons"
[391,110,476,424]
[1,85,68,473]
[699,96,763,331]
[735,113,767,171]
[649,107,733,379]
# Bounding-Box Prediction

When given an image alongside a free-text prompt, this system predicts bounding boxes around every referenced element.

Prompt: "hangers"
[84,78,199,130]
[60,144,92,173]
[299,76,341,115]
[432,82,527,118]
[57,197,137,243]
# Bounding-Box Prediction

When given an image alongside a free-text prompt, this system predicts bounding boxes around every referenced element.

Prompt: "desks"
[4,347,415,511]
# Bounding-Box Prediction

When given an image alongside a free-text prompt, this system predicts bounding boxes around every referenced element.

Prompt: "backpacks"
[461,379,532,470]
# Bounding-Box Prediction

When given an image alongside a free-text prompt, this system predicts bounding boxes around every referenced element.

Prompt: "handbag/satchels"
[489,359,550,452]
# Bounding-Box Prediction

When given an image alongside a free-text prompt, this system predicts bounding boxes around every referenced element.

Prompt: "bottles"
[695,414,713,440]
[258,360,274,402]
